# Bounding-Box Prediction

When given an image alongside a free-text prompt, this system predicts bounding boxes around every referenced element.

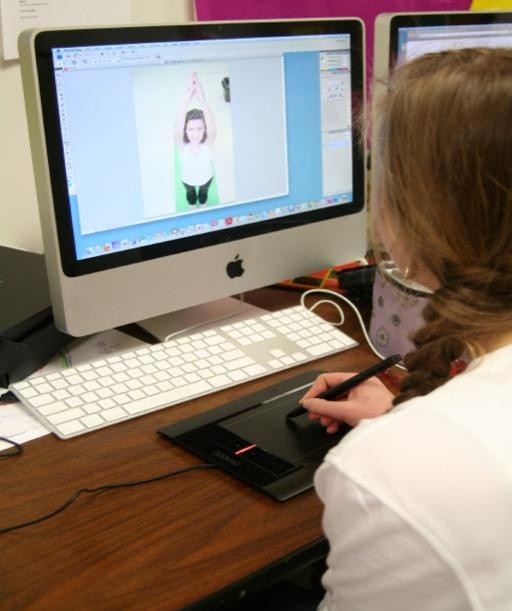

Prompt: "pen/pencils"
[286,353,403,417]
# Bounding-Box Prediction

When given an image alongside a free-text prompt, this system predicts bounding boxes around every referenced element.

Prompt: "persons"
[168,71,220,211]
[299,46,512,611]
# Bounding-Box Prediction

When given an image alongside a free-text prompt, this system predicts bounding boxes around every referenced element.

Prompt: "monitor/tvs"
[17,15,369,341]
[372,11,511,121]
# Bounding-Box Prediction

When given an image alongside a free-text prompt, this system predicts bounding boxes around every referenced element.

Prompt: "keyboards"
[7,304,360,441]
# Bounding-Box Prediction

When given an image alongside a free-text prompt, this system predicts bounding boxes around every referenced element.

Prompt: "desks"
[1,272,412,610]
